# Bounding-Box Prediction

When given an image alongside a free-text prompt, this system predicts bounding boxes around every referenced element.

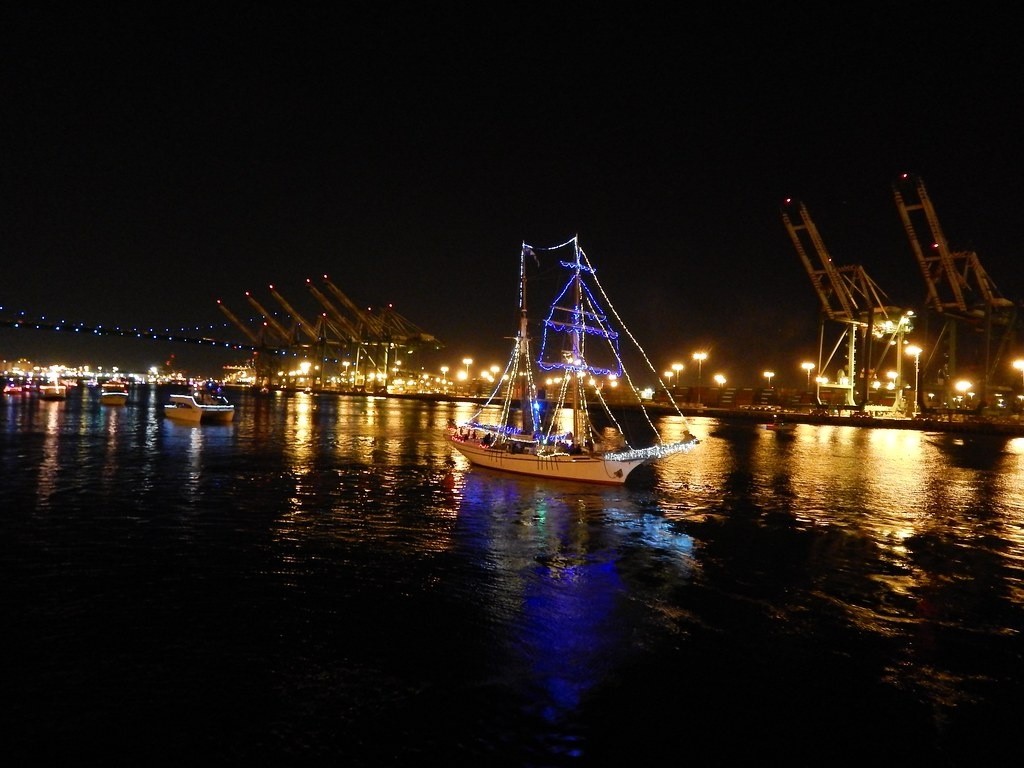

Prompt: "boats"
[260,387,270,395]
[85,372,104,387]
[21,372,37,391]
[101,366,130,405]
[40,369,66,400]
[304,386,312,394]
[163,375,235,424]
[60,377,77,389]
[2,376,21,392]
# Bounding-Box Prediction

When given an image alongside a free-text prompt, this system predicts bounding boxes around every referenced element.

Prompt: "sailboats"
[443,226,703,482]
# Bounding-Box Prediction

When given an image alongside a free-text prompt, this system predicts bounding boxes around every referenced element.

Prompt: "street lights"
[463,358,471,381]
[343,362,350,373]
[1013,358,1024,387]
[672,363,684,385]
[441,367,449,379]
[764,372,775,390]
[905,345,922,413]
[801,361,816,390]
[955,380,973,410]
[887,371,899,386]
[692,352,707,405]
[664,371,674,384]
[715,374,726,388]
